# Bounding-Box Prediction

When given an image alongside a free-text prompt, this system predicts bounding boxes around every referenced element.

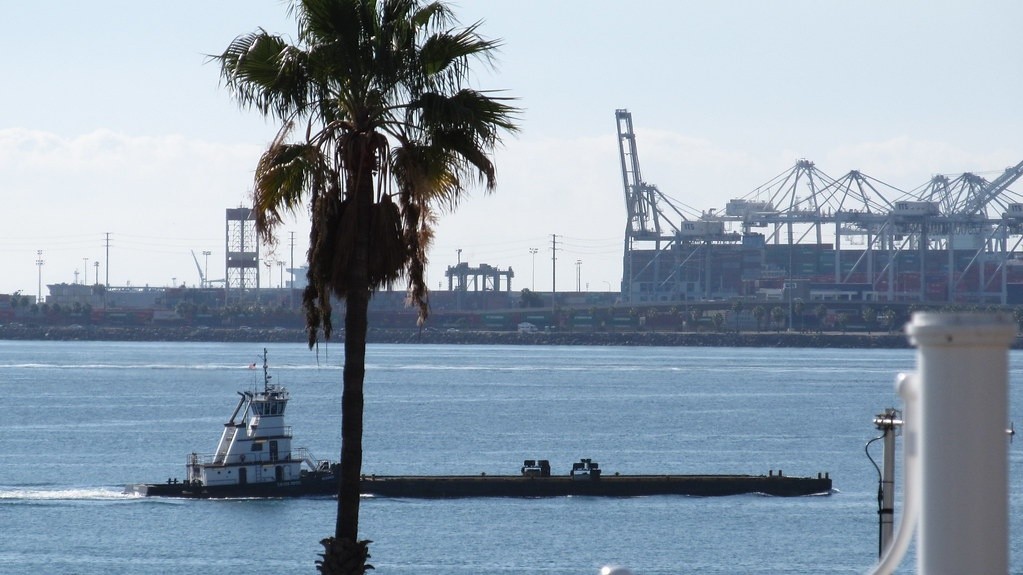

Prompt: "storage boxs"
[620,236,1023,305]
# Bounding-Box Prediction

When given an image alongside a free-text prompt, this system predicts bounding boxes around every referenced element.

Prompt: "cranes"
[615,107,1023,306]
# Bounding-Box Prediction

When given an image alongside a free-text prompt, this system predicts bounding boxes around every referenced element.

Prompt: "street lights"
[277,260,286,289]
[529,247,538,291]
[94,261,99,284]
[202,250,211,289]
[35,249,43,303]
[576,259,582,292]
[82,257,89,285]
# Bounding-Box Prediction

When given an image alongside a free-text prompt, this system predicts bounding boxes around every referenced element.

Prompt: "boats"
[120,348,338,498]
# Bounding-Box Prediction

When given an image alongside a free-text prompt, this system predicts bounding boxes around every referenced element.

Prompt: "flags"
[250,363,256,370]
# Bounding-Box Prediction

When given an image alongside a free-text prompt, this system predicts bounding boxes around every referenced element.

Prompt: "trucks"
[518,323,539,333]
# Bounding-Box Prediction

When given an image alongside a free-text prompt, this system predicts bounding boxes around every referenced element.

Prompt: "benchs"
[570,469,601,478]
[521,467,550,476]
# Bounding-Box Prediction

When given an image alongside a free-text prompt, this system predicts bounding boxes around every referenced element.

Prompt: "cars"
[240,325,251,330]
[424,327,438,332]
[274,327,285,331]
[447,328,459,333]
